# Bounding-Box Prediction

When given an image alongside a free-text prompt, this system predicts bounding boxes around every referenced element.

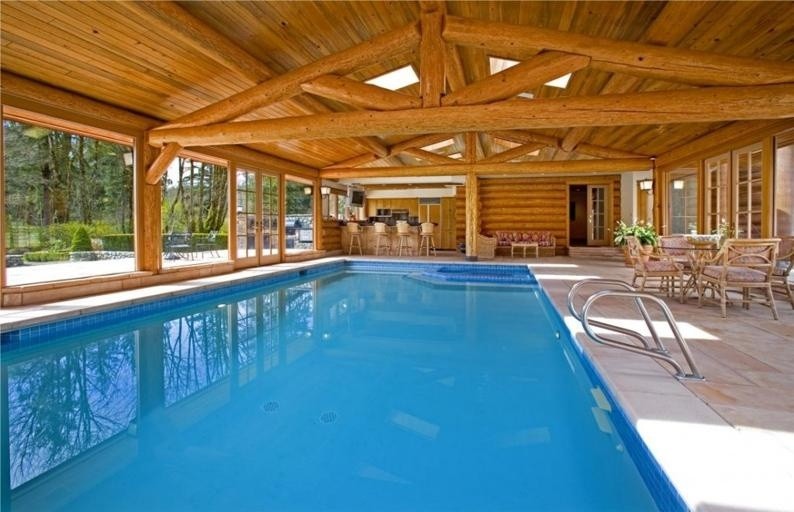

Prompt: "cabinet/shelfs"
[368,199,441,225]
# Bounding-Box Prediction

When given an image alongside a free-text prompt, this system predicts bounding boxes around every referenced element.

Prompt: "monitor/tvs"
[350,189,365,207]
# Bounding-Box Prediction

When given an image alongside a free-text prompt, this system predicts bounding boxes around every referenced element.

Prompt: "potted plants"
[608,220,658,268]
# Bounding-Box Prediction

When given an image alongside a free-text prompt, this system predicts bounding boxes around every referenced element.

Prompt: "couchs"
[490,230,557,257]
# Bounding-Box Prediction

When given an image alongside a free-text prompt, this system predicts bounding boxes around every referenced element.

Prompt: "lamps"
[304,185,313,195]
[321,186,331,199]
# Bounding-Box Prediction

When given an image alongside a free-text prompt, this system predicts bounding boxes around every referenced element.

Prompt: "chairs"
[166,230,224,260]
[418,222,437,257]
[374,222,390,256]
[347,221,363,255]
[624,234,794,321]
[396,221,412,256]
[477,232,498,259]
